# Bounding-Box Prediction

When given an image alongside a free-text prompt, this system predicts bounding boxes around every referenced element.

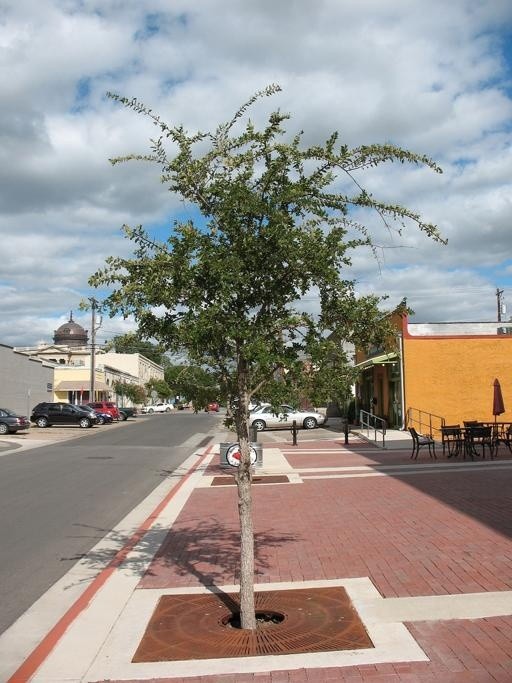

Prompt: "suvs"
[30,402,100,428]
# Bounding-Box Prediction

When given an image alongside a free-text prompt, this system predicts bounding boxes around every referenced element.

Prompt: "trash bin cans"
[237,426,258,442]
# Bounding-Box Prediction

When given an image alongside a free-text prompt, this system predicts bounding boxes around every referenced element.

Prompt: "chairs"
[409,428,437,460]
[441,421,511,462]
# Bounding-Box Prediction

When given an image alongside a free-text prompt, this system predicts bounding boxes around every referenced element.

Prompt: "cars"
[205,402,219,412]
[141,402,173,413]
[0,408,30,435]
[248,402,328,431]
[75,401,137,425]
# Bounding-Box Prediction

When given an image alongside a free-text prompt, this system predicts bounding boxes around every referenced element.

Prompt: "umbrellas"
[491,377,506,426]
